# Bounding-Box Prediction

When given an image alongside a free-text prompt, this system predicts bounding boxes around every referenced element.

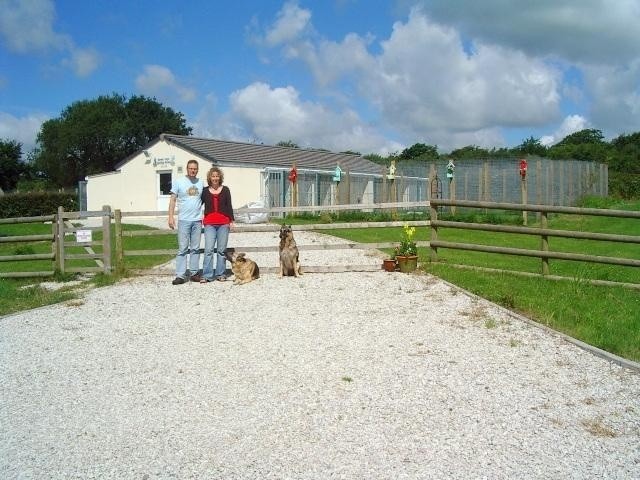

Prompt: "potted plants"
[384,248,397,272]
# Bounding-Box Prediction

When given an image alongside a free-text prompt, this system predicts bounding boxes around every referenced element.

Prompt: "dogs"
[223,250,260,286]
[279,223,303,277]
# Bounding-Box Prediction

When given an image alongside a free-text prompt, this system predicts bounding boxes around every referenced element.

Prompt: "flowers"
[398,224,418,256]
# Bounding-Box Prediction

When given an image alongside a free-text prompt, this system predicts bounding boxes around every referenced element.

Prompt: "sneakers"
[200,277,207,283]
[191,274,200,281]
[217,276,226,281]
[172,277,184,284]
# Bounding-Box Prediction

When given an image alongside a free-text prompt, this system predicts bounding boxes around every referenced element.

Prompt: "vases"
[396,255,418,273]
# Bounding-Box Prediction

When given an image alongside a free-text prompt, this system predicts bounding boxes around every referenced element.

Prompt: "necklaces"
[211,185,220,190]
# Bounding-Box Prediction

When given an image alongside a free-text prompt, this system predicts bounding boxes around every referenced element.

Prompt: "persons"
[167,159,205,285]
[200,166,235,285]
